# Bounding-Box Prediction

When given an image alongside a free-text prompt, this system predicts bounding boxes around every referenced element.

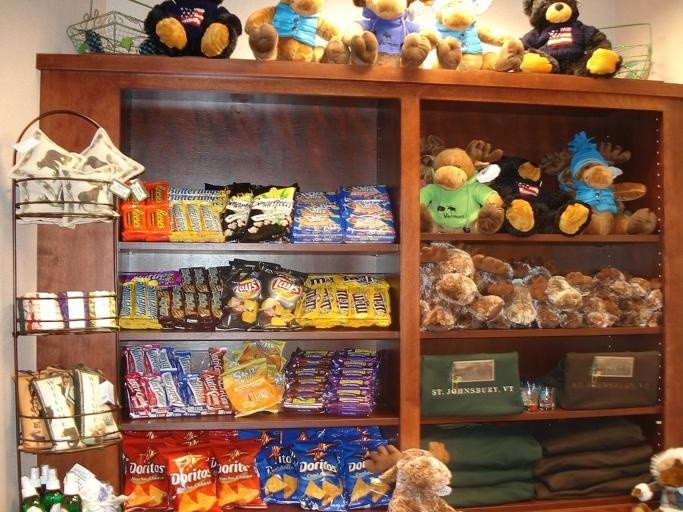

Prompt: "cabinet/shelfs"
[36,54,683,512]
[12,109,123,512]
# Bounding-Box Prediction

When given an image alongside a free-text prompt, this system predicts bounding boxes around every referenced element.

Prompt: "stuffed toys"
[631,447,683,512]
[365,440,459,512]
[513,0,623,79]
[420,129,663,333]
[421,0,524,72]
[245,0,352,64]
[341,0,439,69]
[419,242,664,333]
[144,1,242,59]
[421,130,662,236]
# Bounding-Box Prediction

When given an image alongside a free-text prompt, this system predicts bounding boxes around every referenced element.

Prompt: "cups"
[522,386,556,410]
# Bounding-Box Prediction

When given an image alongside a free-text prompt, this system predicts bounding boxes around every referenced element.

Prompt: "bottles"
[21,463,81,512]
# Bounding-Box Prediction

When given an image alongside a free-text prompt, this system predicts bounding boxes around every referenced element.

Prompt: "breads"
[419,240,663,331]
